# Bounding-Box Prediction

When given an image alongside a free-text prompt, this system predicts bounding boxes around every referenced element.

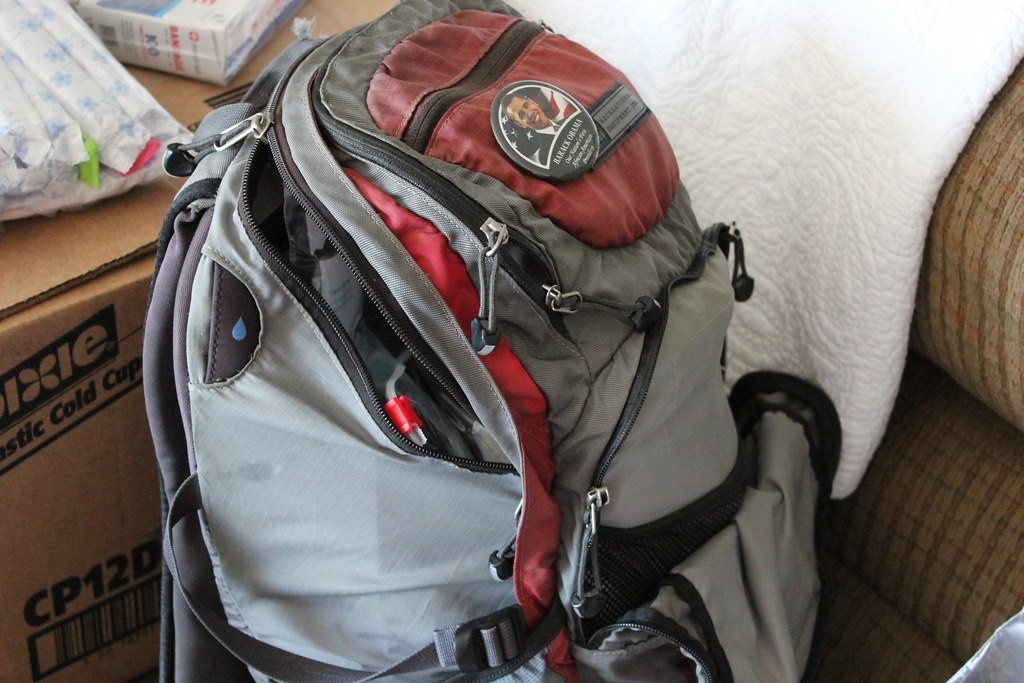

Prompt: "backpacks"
[142,0,843,683]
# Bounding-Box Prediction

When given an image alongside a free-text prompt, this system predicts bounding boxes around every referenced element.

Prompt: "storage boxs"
[0,0,396,683]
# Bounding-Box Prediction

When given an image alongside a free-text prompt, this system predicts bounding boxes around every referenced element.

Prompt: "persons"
[501,93,570,166]
